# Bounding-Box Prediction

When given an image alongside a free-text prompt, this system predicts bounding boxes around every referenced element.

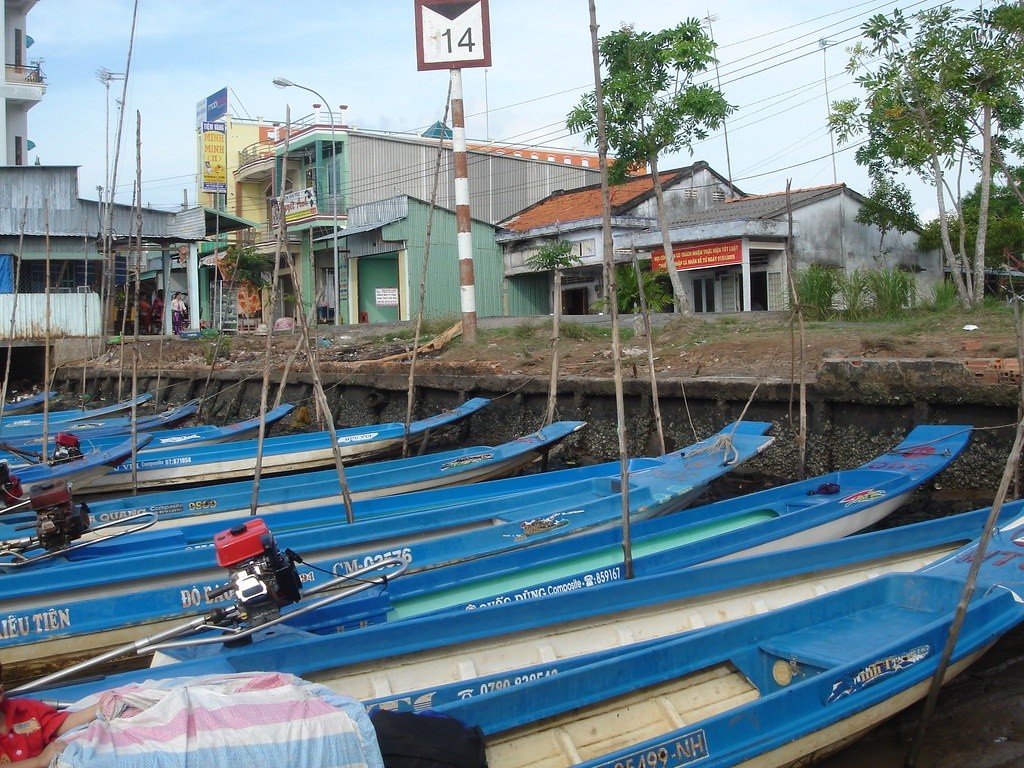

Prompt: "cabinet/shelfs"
[210,280,240,335]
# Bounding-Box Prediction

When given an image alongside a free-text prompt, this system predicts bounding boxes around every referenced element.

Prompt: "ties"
[177,302,181,311]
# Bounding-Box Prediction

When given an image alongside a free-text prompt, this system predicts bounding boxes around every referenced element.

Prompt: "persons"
[140,289,186,335]
[0,662,115,768]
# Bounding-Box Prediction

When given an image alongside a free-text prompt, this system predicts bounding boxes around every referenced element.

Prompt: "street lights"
[271,77,341,332]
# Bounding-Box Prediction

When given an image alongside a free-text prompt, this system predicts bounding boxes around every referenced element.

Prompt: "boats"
[0,107,1024,768]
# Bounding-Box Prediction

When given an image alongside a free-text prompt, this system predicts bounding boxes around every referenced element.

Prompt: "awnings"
[311,217,404,242]
